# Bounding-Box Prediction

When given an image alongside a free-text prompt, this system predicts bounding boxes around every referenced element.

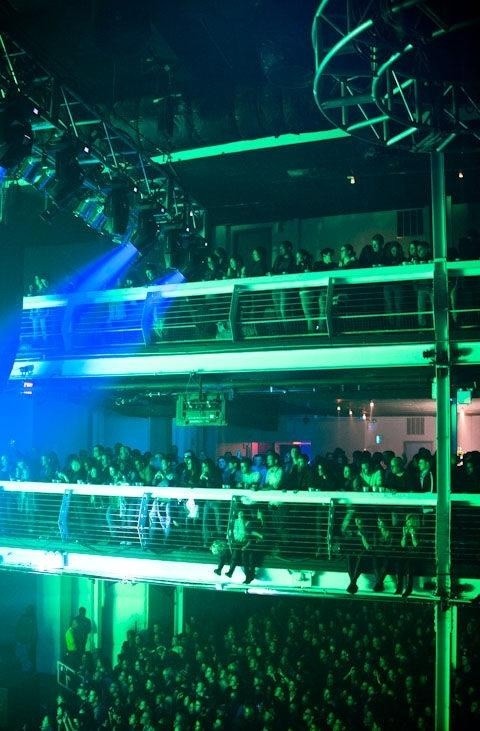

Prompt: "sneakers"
[346,582,410,598]
[214,568,255,583]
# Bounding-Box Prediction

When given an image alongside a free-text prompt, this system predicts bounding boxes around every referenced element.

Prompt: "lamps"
[0,29,211,281]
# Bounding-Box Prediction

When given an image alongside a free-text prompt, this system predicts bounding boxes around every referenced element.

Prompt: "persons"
[23,231,459,345]
[13,595,480,731]
[1,438,480,600]
